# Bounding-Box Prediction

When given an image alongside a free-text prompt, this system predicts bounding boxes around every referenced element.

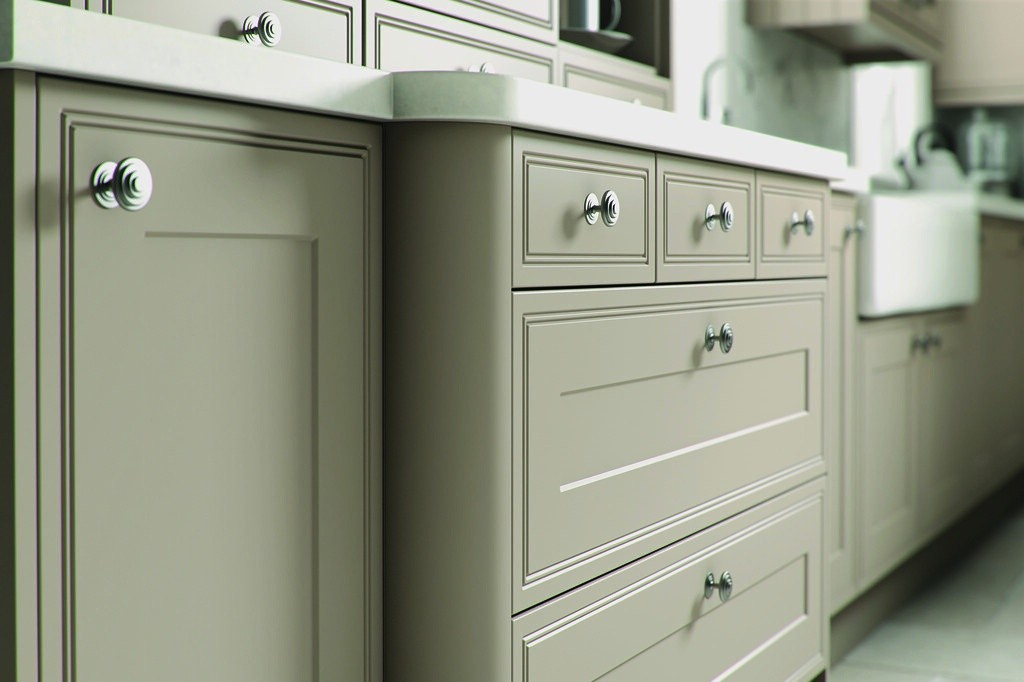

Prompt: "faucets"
[699,47,757,123]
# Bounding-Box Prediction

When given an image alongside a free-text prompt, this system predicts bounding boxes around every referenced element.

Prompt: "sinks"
[856,189,982,319]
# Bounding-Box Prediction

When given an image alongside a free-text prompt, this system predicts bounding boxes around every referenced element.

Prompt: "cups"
[559,0,622,30]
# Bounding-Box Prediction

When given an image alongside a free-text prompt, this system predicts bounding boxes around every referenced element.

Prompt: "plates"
[559,28,634,54]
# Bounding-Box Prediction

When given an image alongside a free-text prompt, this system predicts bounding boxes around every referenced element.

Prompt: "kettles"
[898,123,967,190]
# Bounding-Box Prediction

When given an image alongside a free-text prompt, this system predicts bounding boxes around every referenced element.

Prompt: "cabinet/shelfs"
[0,1,1024,679]
